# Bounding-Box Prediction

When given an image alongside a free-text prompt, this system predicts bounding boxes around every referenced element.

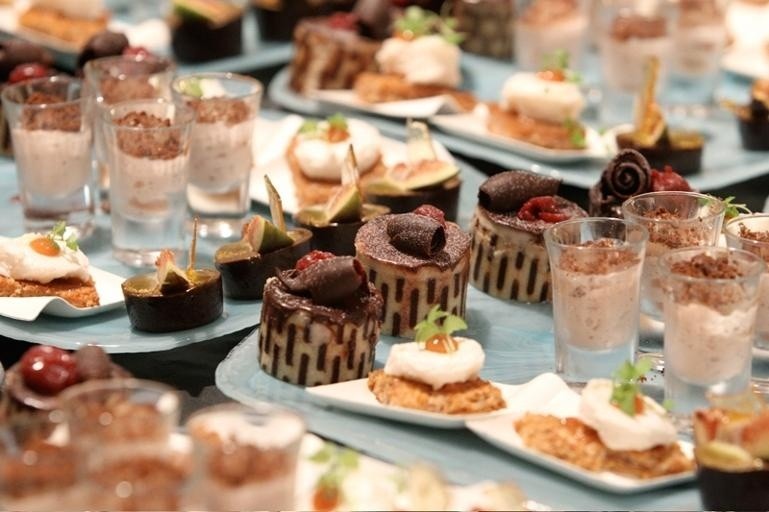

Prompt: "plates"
[309,87,611,165]
[307,377,538,429]
[466,380,701,494]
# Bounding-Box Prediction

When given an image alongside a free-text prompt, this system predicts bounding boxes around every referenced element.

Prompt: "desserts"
[1,0,769,512]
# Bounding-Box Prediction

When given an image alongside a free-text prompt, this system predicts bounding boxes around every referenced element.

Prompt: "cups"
[694,447,769,511]
[513,1,733,129]
[726,214,768,385]
[653,246,766,415]
[167,72,263,218]
[85,56,177,205]
[96,100,198,251]
[546,218,649,391]
[3,378,306,511]
[621,189,728,353]
[1,77,98,226]
